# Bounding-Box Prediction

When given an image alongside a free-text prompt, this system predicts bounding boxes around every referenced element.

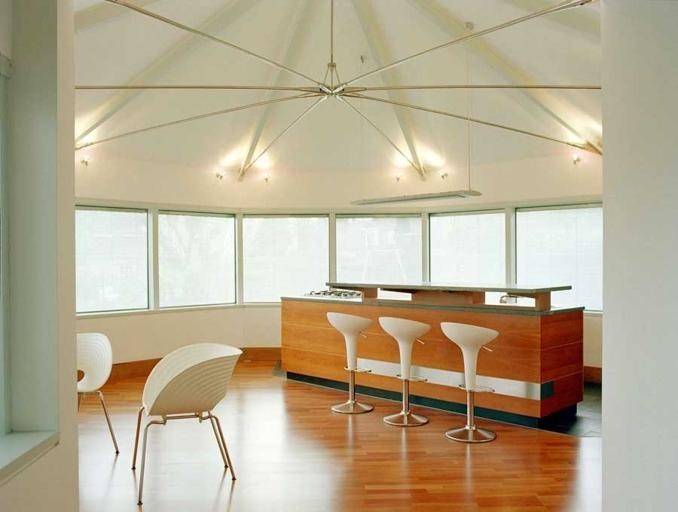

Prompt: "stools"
[326,308,501,444]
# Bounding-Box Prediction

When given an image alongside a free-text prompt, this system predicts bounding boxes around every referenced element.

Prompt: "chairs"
[74,331,121,458]
[129,342,243,506]
